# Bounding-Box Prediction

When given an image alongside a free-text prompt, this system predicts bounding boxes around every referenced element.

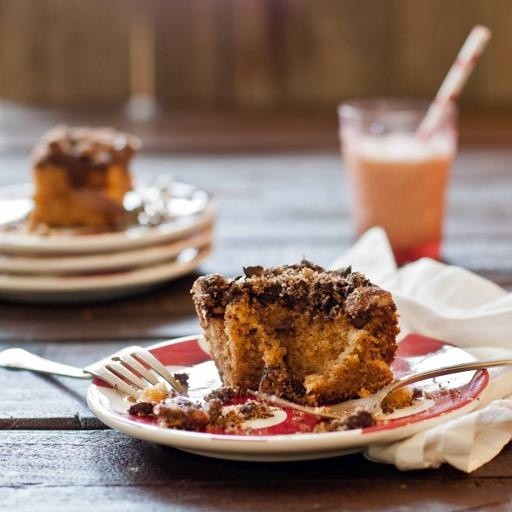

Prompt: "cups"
[333,97,462,261]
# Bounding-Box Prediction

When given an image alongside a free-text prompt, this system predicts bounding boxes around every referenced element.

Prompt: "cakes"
[189,259,401,407]
[30,124,142,232]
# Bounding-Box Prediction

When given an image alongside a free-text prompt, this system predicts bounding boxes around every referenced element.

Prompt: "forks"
[246,360,512,418]
[0,346,191,401]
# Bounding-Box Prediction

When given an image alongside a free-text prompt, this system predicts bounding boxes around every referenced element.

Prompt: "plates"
[85,330,502,466]
[0,179,217,303]
